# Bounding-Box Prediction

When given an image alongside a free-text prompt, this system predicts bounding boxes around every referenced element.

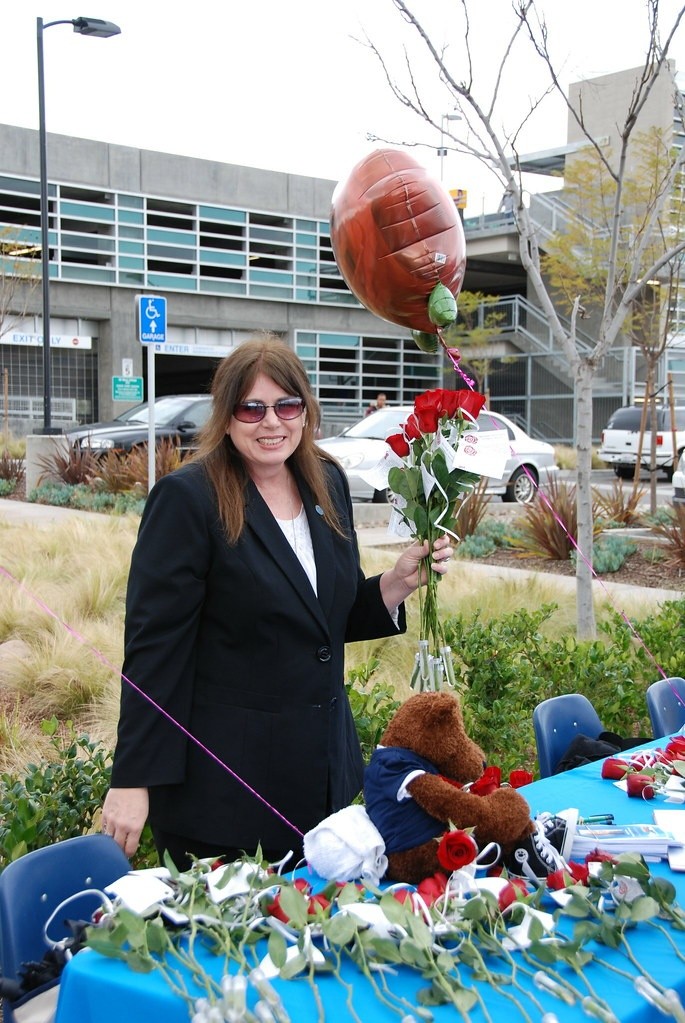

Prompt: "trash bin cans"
[25,435,69,498]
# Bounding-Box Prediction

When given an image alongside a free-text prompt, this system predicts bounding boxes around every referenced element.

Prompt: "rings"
[105,831,113,838]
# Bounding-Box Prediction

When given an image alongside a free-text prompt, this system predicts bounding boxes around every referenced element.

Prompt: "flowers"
[80,735,685,1023]
[387,388,488,694]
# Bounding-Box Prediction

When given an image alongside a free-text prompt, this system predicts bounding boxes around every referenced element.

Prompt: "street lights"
[441,114,462,182]
[29,16,123,435]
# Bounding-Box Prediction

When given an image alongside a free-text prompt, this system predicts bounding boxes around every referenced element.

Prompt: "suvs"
[597,406,685,480]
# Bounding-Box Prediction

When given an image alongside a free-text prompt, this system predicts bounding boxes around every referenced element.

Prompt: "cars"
[63,393,214,472]
[311,405,560,504]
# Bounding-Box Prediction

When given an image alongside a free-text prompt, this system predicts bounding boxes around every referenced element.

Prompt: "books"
[571,824,685,863]
[653,810,685,871]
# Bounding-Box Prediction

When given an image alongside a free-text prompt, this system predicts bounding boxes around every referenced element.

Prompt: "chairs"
[532,693,605,780]
[645,676,685,739]
[0,832,134,1023]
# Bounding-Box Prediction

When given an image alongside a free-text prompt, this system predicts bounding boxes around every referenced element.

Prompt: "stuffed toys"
[363,690,578,886]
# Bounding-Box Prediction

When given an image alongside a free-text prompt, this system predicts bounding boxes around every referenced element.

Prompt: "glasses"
[231,397,306,423]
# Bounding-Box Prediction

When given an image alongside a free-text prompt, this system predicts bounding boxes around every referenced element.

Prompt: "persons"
[366,393,386,418]
[101,331,455,877]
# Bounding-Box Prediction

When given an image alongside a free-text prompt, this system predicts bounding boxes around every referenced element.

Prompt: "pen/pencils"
[577,814,615,824]
[584,820,612,825]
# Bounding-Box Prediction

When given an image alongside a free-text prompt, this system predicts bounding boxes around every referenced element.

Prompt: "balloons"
[333,149,467,353]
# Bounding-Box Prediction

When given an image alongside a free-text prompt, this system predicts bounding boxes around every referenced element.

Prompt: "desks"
[53,729,685,1023]
